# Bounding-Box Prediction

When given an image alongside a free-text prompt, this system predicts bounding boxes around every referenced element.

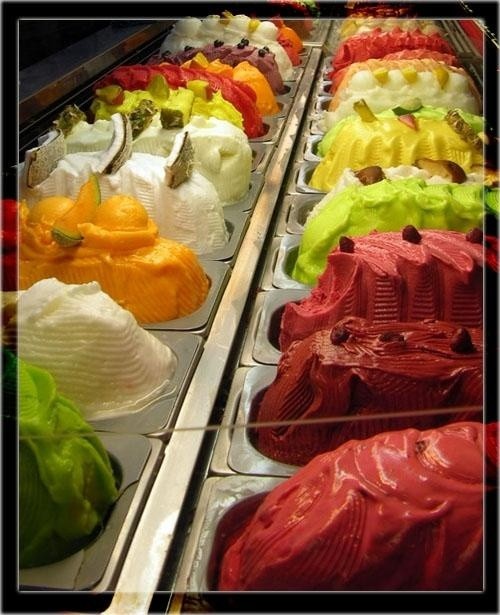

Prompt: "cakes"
[210,0,499,591]
[17,20,304,576]
